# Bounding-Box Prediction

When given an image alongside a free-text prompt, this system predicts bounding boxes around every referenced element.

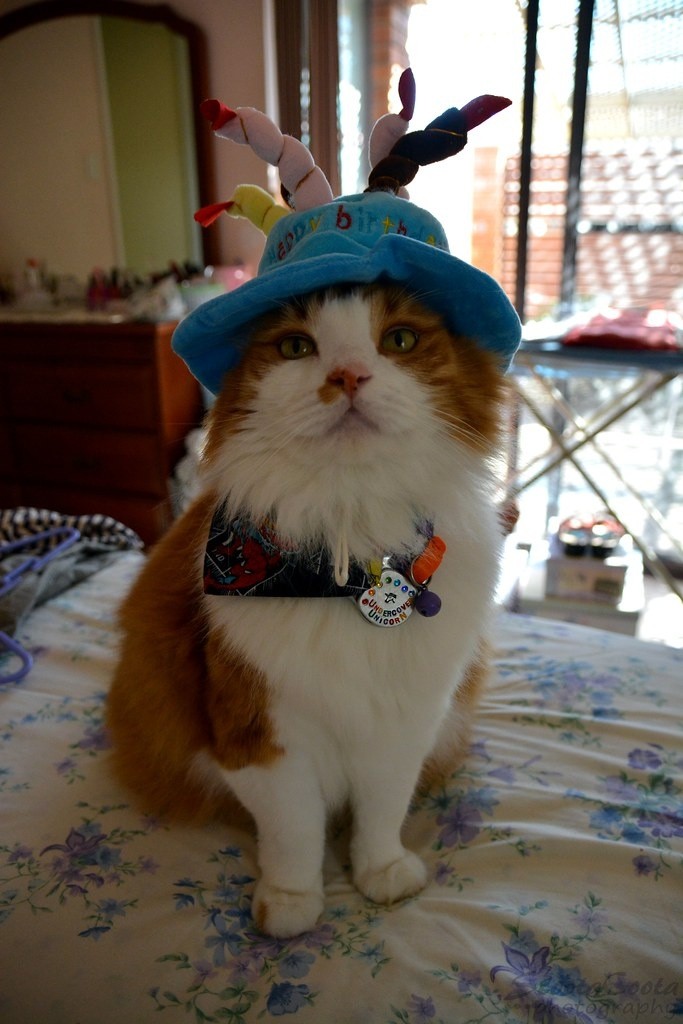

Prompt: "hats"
[171,66,525,386]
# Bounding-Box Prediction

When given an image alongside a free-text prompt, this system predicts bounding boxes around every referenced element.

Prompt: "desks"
[500,311,683,603]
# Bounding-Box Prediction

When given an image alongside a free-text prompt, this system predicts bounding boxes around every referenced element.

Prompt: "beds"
[0,552,682,1024]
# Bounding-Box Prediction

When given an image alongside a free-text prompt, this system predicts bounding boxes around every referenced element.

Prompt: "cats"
[105,284,514,941]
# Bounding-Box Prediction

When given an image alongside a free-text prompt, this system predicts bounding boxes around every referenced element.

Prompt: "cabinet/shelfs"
[1,17,205,554]
